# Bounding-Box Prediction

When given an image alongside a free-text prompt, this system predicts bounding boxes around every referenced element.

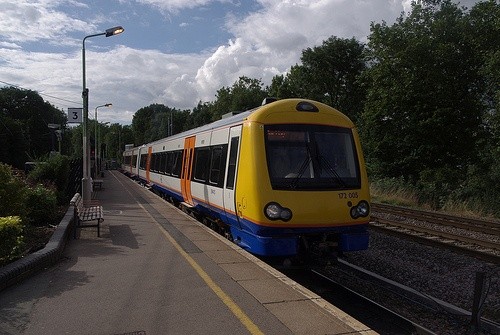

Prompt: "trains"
[121,97,374,268]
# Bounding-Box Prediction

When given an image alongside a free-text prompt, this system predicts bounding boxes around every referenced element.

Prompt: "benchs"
[70,192,104,237]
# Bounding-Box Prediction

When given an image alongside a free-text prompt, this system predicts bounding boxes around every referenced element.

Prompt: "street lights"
[48,123,61,154]
[82,26,124,206]
